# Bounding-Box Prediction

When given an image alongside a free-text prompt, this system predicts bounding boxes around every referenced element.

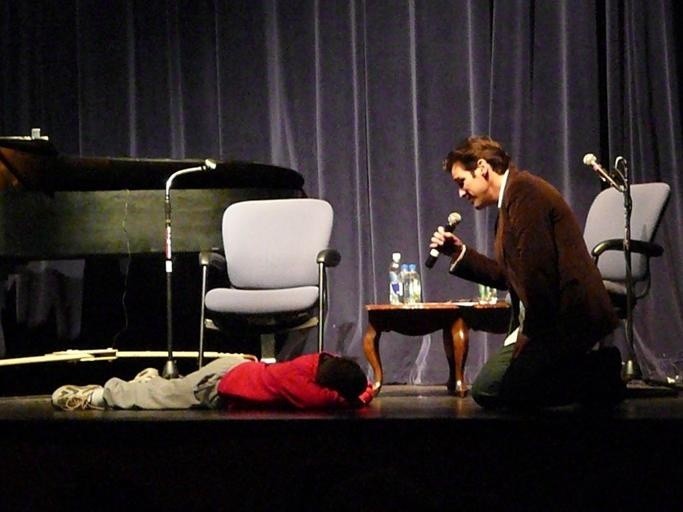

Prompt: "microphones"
[424,212,462,270]
[581,152,625,196]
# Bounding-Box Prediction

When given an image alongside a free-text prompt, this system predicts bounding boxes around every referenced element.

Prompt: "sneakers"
[585,344,626,410]
[51,384,107,411]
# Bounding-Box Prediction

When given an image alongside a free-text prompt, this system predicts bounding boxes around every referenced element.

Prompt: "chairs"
[580,182,669,378]
[194,197,340,371]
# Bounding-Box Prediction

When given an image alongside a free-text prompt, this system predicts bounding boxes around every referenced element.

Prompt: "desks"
[358,303,511,399]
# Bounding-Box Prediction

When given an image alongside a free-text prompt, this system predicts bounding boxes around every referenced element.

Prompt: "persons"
[426,131,629,413]
[47,350,377,414]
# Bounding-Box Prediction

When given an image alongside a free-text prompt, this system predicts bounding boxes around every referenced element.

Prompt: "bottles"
[388,252,403,305]
[400,262,422,304]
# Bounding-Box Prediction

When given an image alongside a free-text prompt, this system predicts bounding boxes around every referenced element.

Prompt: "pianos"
[0,135,307,397]
